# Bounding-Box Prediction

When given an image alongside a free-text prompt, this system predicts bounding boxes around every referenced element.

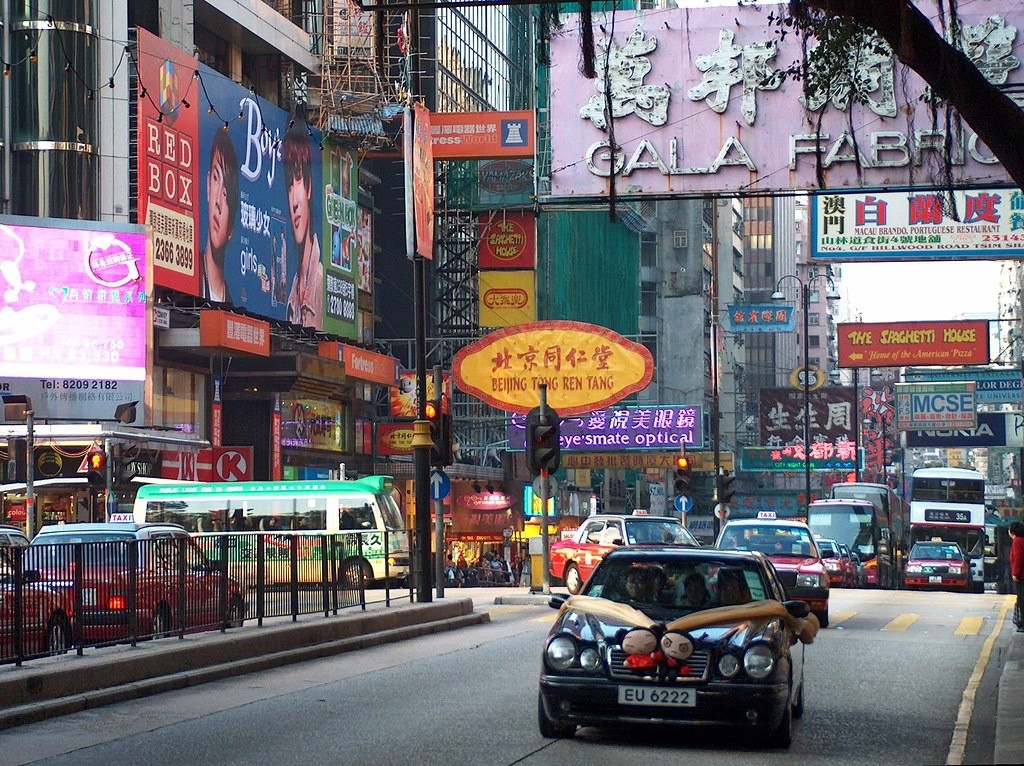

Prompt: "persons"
[680,574,708,607]
[238,511,282,530]
[342,504,365,528]
[445,550,531,587]
[285,115,322,331]
[298,517,309,529]
[1008,522,1024,632]
[919,549,927,557]
[617,567,652,606]
[199,128,240,304]
[728,528,748,549]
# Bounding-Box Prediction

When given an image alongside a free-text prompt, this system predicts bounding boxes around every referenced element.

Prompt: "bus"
[827,482,909,583]
[806,499,891,591]
[131,475,412,588]
[909,467,985,593]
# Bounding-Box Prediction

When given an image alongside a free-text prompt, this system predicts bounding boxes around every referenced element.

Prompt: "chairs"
[166,517,298,531]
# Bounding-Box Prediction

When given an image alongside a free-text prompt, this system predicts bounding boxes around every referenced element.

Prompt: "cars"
[537,546,806,748]
[812,534,867,591]
[904,535,972,591]
[709,511,831,629]
[0,521,246,664]
[546,507,704,596]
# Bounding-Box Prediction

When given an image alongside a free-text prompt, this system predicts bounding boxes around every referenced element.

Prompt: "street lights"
[770,274,843,527]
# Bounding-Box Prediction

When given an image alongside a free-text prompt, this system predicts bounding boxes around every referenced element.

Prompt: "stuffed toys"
[615,625,660,680]
[651,623,708,681]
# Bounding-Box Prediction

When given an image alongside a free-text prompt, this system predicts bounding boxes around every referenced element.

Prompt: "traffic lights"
[86,451,109,492]
[528,419,561,472]
[424,399,444,441]
